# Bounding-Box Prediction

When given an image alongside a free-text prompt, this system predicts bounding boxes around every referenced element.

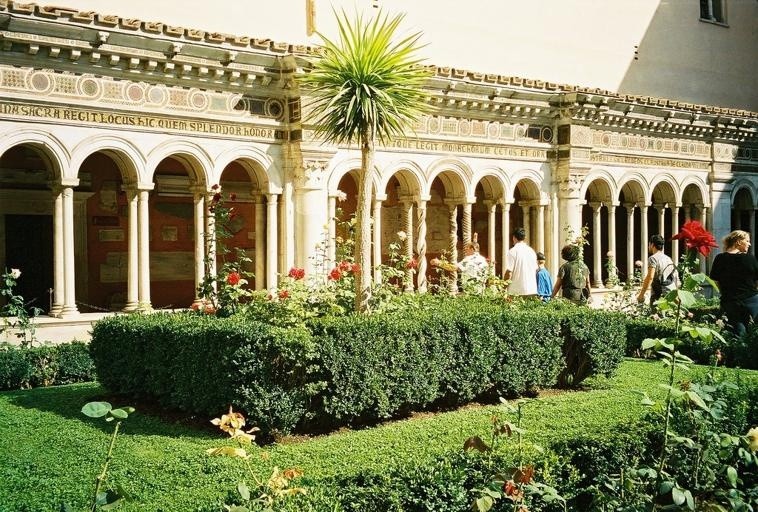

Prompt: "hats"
[537,252,545,260]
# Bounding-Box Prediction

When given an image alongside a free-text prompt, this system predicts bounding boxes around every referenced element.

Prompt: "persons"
[502,226,539,300]
[637,233,678,308]
[549,243,591,301]
[535,252,553,303]
[709,229,758,321]
[453,242,489,295]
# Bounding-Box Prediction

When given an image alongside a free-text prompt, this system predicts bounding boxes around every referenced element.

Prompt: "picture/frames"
[98,251,195,283]
[186,222,194,235]
[239,247,255,279]
[98,229,126,243]
[149,224,154,242]
[92,214,121,227]
[119,201,129,219]
[160,223,179,243]
